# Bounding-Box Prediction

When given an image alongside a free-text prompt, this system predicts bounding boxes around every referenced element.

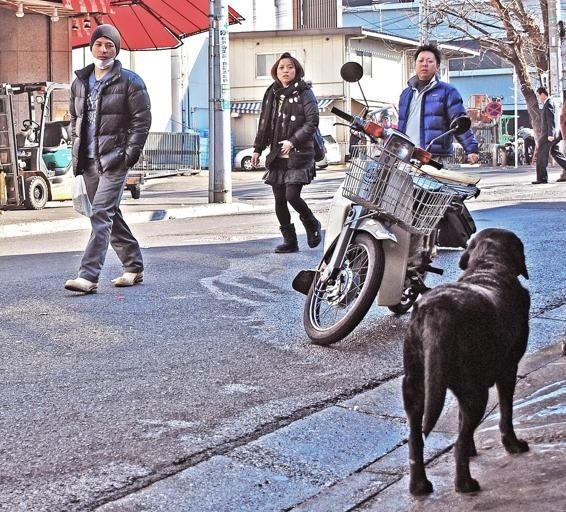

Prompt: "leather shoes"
[532,180,547,183]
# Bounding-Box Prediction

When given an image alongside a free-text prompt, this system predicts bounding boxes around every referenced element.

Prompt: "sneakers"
[64,278,98,294]
[557,179,566,181]
[115,271,144,287]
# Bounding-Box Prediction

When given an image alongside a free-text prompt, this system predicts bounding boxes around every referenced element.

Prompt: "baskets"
[342,143,456,237]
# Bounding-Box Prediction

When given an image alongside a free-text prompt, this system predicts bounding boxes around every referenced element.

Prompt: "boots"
[275,224,299,253]
[300,213,322,248]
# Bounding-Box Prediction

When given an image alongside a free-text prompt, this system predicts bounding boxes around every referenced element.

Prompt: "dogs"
[401,227,531,496]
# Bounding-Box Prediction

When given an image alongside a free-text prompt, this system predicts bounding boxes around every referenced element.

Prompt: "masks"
[90,54,114,71]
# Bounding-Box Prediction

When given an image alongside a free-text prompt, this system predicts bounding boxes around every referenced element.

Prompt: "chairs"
[38,121,68,167]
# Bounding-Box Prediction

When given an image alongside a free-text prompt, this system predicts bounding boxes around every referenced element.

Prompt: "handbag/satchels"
[312,130,327,163]
[72,194,93,217]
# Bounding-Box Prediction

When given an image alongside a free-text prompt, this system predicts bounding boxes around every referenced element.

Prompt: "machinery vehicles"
[0,80,146,213]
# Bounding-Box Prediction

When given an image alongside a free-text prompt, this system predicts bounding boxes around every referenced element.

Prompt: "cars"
[234,133,345,172]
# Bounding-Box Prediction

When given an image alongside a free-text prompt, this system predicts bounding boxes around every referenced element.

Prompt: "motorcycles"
[289,61,483,349]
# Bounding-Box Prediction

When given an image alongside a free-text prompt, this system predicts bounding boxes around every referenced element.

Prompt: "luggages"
[436,194,476,248]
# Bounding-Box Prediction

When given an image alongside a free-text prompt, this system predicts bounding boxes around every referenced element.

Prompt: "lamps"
[14,1,102,30]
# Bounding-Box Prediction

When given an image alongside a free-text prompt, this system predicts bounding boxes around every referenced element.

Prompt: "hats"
[90,24,121,57]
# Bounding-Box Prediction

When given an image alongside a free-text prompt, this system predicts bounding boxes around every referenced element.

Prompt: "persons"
[399,44,480,175]
[252,52,328,255]
[63,22,151,293]
[532,80,566,187]
